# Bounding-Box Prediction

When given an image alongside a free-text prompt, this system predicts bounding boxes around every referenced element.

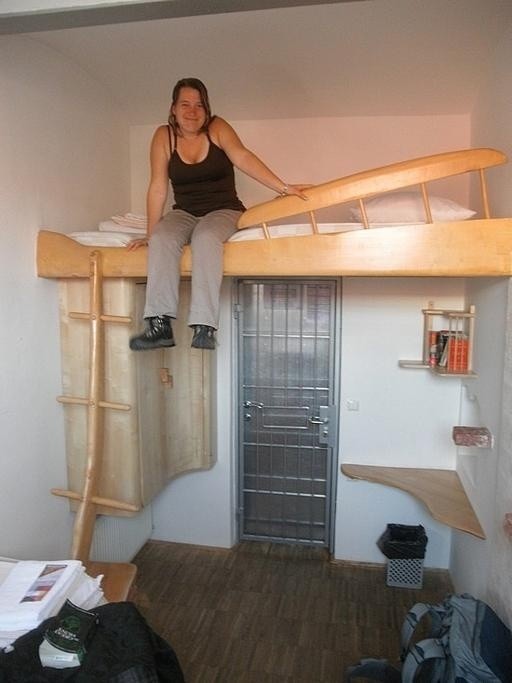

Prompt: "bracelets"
[281,184,290,197]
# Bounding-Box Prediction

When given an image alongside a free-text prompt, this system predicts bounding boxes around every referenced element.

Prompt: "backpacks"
[401,592,512,683]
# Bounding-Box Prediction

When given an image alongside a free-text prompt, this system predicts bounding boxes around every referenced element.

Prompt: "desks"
[0,559,139,682]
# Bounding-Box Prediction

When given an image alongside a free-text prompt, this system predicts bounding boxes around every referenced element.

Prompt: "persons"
[126,78,313,351]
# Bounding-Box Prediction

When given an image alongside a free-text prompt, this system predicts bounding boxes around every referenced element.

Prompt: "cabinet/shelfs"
[64,278,217,518]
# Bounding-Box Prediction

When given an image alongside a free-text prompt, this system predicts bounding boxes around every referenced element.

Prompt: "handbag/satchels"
[0,599,184,682]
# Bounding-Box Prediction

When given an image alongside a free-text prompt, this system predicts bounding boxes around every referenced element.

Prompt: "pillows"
[348,190,478,222]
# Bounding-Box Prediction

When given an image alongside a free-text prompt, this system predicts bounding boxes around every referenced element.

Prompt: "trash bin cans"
[386,524,426,589]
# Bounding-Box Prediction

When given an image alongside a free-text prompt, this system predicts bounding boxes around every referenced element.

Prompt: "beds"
[36,148,511,284]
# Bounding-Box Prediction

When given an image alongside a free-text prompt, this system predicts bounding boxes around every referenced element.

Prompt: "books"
[428,330,468,372]
[38,597,104,670]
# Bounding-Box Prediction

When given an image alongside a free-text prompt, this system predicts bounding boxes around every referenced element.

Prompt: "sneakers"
[130,316,175,350]
[191,326,216,349]
